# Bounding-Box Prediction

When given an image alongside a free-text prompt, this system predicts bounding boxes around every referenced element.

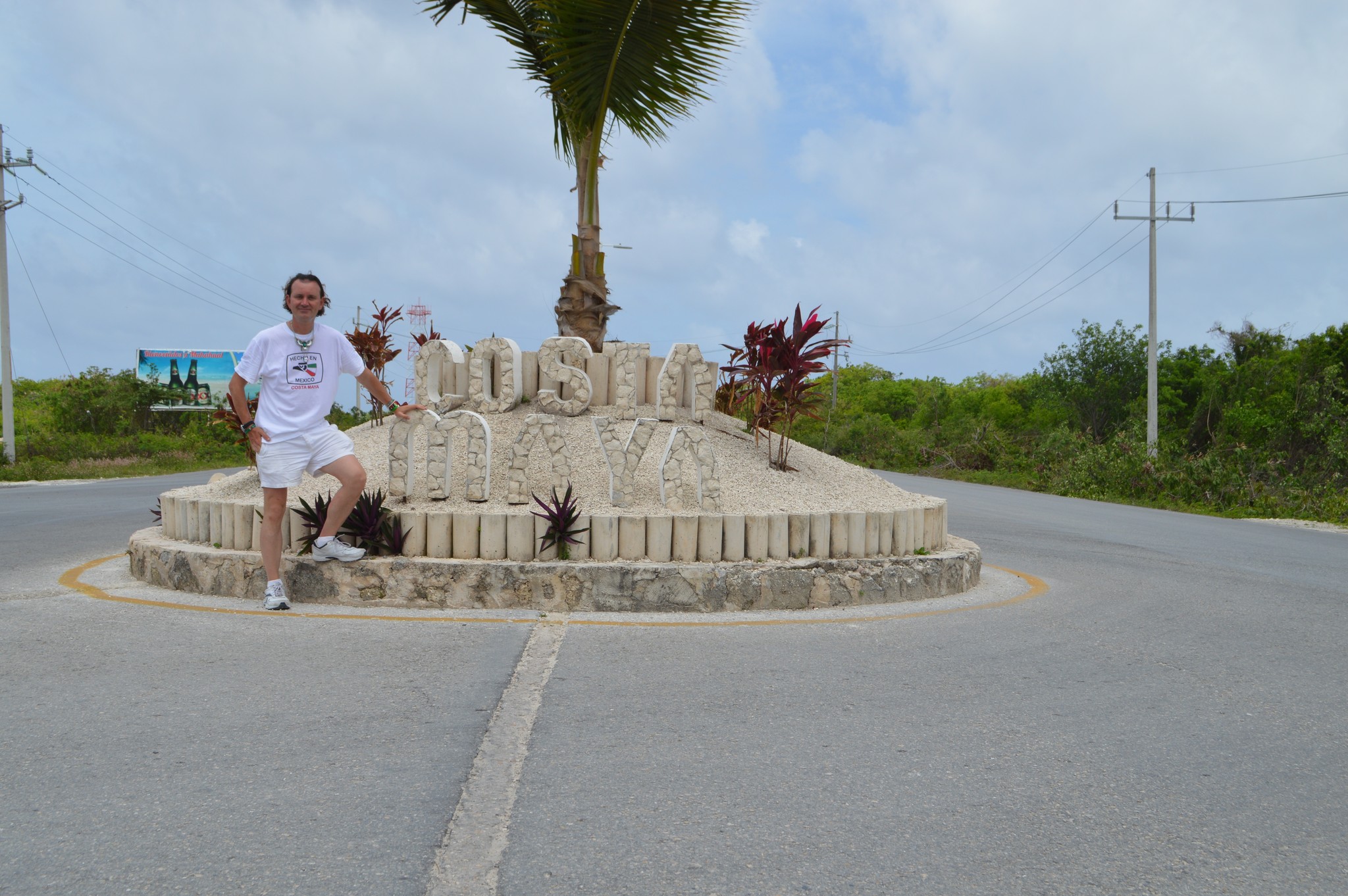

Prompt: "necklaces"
[290,319,315,363]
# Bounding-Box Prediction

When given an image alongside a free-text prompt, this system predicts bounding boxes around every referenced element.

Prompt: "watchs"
[240,421,255,433]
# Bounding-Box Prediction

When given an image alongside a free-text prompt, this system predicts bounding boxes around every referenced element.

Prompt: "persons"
[228,274,427,610]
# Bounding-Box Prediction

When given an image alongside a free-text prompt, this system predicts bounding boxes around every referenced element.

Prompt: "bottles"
[168,359,184,406]
[184,359,199,406]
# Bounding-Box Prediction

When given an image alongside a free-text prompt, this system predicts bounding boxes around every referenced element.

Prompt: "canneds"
[155,383,171,407]
[197,383,212,407]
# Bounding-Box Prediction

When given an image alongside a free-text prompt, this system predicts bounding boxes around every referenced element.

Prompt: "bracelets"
[244,424,256,434]
[385,399,399,413]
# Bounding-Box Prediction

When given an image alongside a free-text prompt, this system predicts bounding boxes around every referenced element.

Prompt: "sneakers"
[263,582,290,610]
[312,534,367,562]
